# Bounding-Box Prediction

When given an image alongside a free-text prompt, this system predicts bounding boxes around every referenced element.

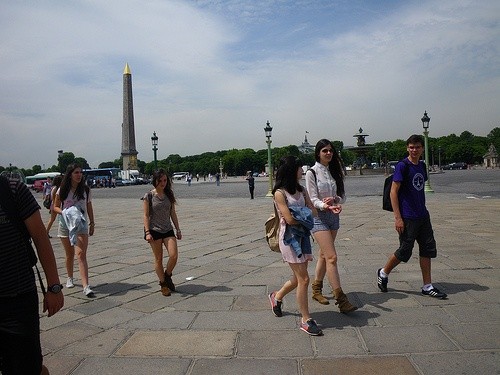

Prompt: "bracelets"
[145,230,150,236]
[89,221,95,226]
[176,229,181,233]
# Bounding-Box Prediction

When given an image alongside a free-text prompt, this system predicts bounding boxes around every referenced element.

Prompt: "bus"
[25,172,64,189]
[83,168,123,186]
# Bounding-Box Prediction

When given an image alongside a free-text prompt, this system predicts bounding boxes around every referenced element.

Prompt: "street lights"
[264,120,273,196]
[150,131,158,171]
[421,110,434,192]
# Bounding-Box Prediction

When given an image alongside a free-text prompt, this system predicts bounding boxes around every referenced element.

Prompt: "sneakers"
[83,285,93,296]
[66,278,74,288]
[300,318,322,334]
[422,286,447,299]
[376,268,389,292]
[269,292,282,317]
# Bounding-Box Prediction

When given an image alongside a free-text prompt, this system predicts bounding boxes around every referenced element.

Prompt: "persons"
[377,135,447,300]
[54,162,96,296]
[482,143,496,157]
[0,174,64,375]
[143,170,181,296]
[43,176,62,238]
[305,139,357,314]
[246,173,254,199]
[87,177,115,188]
[268,156,322,335]
[186,173,221,186]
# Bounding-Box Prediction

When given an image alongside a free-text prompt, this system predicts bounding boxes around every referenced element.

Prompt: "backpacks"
[265,203,281,252]
[43,194,50,209]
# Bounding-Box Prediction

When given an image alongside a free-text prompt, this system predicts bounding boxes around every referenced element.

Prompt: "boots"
[165,270,175,291]
[312,280,330,305]
[159,281,170,296]
[331,287,357,313]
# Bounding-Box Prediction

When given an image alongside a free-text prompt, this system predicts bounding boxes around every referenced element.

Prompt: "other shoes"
[48,236,52,238]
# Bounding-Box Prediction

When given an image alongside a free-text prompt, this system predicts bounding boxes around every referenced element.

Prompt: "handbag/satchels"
[383,160,408,212]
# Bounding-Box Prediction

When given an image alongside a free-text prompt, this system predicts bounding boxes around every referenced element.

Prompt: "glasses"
[319,149,333,153]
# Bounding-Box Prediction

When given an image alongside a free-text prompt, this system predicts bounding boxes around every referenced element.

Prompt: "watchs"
[47,285,63,294]
